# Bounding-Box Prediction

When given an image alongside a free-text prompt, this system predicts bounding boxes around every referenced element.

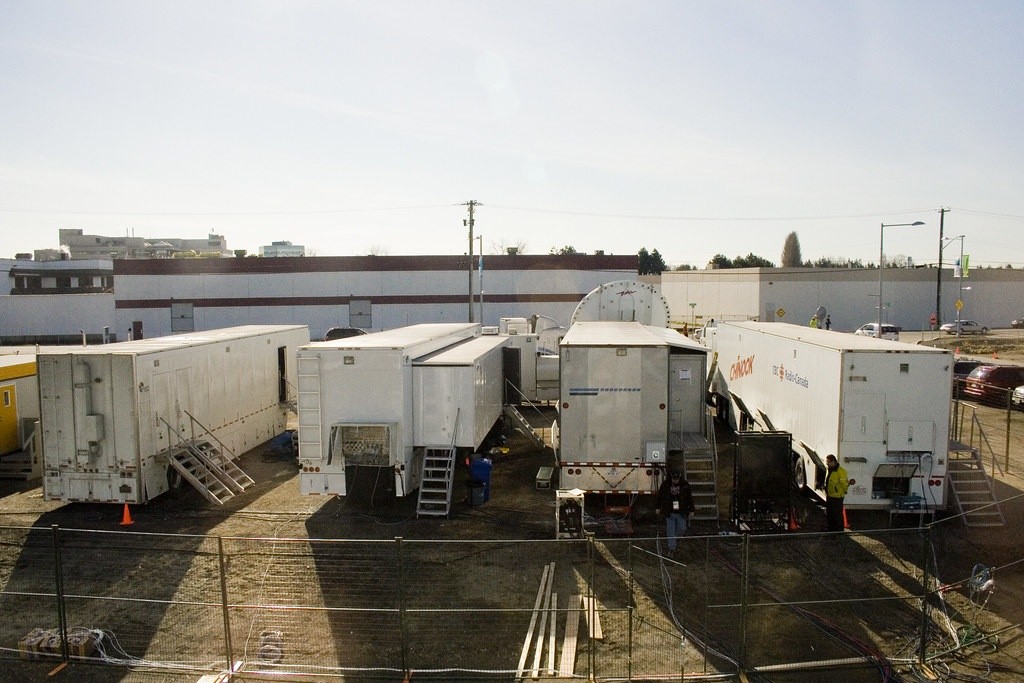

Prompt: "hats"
[671,471,679,480]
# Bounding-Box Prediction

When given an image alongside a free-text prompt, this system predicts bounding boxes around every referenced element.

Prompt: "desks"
[887,506,935,532]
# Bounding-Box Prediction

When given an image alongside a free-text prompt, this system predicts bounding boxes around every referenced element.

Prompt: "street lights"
[878,219,927,342]
[937,235,964,336]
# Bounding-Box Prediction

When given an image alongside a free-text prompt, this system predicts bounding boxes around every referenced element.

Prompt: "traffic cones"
[119,502,134,525]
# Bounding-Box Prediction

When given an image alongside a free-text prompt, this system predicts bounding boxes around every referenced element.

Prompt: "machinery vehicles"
[560,321,721,532]
[700,321,1007,526]
[35,324,310,509]
[298,321,509,517]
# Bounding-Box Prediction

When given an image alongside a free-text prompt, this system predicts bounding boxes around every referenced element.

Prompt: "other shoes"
[668,549,675,557]
[677,540,683,548]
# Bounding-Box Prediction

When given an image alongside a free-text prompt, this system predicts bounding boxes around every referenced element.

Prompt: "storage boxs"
[536,465,555,491]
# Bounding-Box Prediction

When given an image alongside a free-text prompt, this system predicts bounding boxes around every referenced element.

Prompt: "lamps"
[617,348,628,357]
[342,356,355,365]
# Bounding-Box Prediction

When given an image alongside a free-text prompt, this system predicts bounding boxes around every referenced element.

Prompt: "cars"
[855,323,899,343]
[950,358,989,395]
[1011,316,1024,329]
[965,363,1024,406]
[941,321,993,335]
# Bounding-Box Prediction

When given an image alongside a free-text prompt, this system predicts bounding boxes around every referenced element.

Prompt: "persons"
[824,455,848,532]
[656,470,694,557]
[810,314,831,330]
[684,322,688,337]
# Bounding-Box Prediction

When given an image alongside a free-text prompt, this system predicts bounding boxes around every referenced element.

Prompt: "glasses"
[672,478,679,480]
